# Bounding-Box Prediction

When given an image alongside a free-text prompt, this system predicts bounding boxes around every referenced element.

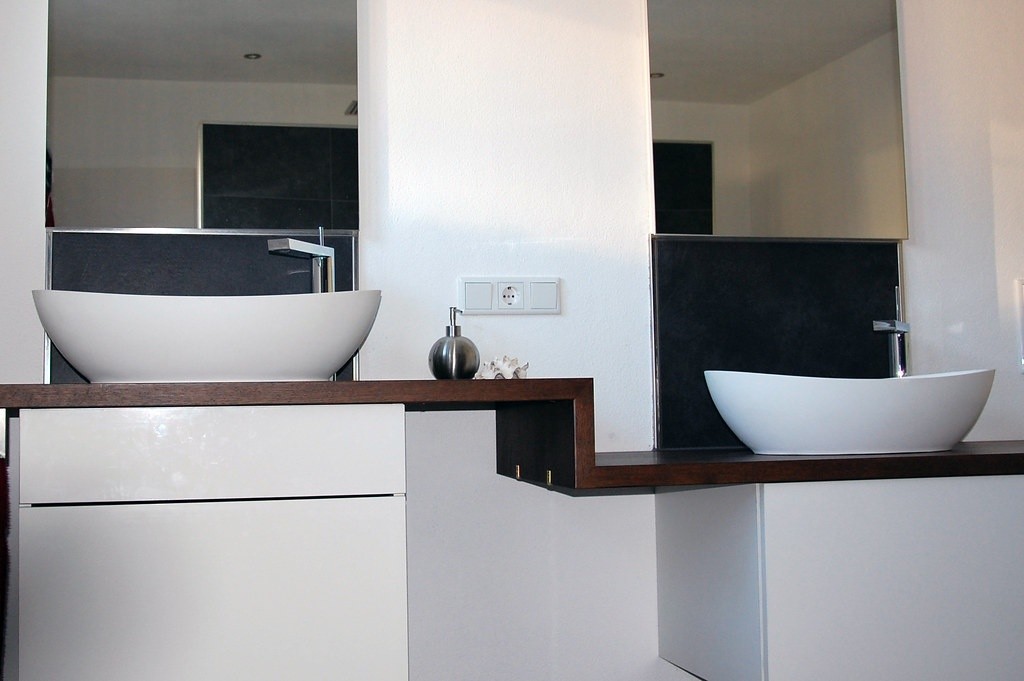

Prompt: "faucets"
[872,318,911,378]
[266,238,336,294]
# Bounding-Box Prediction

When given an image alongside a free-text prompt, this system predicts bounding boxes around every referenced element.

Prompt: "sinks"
[702,368,998,454]
[31,287,383,382]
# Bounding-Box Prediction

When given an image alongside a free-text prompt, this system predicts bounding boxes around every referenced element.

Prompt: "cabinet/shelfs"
[17,404,409,681]
[656,475,1024,681]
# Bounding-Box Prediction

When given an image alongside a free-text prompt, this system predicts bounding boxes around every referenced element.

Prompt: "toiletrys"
[428,305,482,381]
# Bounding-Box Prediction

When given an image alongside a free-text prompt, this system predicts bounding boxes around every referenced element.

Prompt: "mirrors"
[646,0,908,241]
[45,0,359,231]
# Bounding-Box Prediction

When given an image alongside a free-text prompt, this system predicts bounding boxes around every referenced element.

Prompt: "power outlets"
[458,277,560,314]
